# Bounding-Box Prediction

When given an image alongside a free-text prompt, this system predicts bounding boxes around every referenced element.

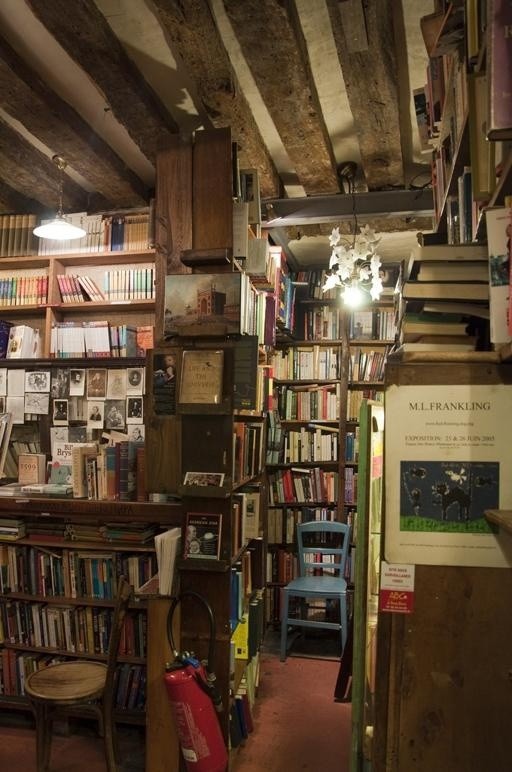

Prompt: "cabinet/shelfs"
[349,349,512,772]
[431,0,512,244]
[0,246,158,370]
[0,492,178,728]
[273,288,403,633]
[183,119,292,754]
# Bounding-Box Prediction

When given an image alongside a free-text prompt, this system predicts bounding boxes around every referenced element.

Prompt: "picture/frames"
[178,348,225,407]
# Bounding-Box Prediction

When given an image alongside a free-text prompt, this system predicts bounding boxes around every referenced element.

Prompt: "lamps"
[32,154,86,241]
[320,161,383,309]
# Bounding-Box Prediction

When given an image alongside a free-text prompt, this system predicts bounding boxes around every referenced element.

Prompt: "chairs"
[278,520,352,666]
[24,575,134,772]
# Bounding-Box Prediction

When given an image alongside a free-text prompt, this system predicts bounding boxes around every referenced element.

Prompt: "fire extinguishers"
[161,590,229,772]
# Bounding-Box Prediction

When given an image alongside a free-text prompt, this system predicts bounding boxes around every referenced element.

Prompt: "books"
[233,212,403,485]
[0,439,150,503]
[1,212,154,258]
[1,320,156,359]
[230,467,358,747]
[385,243,496,353]
[412,1,512,212]
[2,268,154,306]
[1,514,183,712]
